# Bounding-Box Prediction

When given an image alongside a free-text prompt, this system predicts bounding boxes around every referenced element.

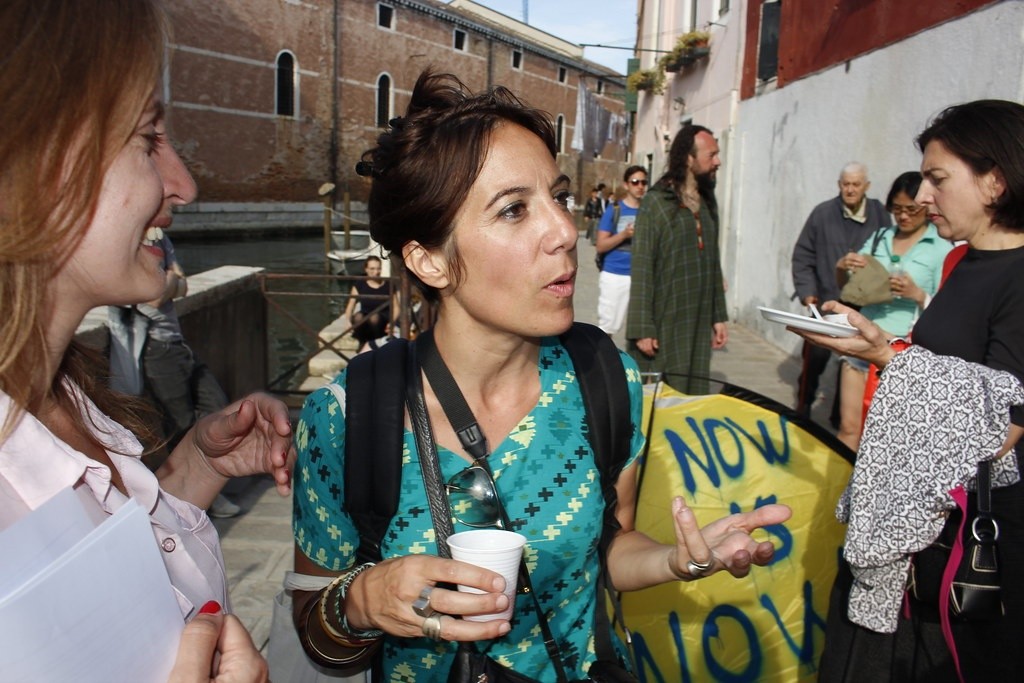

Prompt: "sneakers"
[208,493,240,517]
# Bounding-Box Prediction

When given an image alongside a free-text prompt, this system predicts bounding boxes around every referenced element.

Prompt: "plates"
[758,306,861,338]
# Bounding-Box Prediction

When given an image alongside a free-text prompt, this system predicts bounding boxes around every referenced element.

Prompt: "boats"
[325,229,393,283]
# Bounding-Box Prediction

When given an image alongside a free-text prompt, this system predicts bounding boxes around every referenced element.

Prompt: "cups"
[445,529,527,623]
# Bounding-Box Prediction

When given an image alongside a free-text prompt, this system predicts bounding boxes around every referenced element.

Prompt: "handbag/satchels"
[447,644,637,683]
[900,460,1005,624]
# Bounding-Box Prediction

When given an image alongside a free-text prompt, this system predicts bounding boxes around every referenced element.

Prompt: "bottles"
[888,255,906,301]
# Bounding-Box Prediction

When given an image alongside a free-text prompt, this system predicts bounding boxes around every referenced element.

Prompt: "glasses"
[443,466,530,597]
[889,205,926,216]
[628,179,648,185]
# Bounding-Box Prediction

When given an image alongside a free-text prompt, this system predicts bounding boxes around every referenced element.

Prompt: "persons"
[584,184,615,240]
[0,0,296,683]
[785,99,1024,683]
[626,125,729,393]
[597,165,650,339]
[108,229,250,518]
[834,171,954,454]
[284,72,792,683]
[345,254,430,350]
[791,163,892,430]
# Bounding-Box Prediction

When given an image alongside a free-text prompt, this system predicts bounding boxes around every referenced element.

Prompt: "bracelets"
[888,337,904,345]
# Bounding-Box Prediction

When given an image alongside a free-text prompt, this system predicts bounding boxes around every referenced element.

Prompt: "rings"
[412,585,435,618]
[687,559,714,576]
[421,613,442,643]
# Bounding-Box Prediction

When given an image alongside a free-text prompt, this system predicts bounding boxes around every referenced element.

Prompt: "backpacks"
[595,202,619,272]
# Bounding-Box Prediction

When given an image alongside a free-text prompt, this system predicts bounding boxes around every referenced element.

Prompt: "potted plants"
[626,30,710,94]
